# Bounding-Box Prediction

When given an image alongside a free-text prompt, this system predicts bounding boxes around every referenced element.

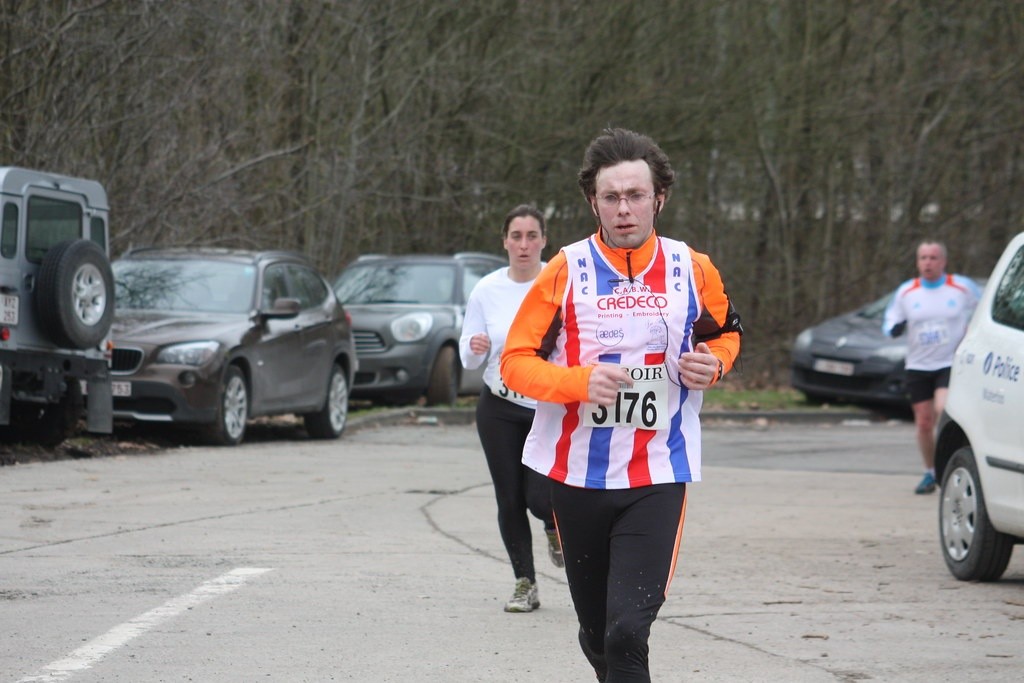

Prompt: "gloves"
[891,320,906,336]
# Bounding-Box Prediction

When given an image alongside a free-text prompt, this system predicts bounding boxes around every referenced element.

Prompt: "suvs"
[328,251,506,404]
[102,243,363,444]
[0,166,117,448]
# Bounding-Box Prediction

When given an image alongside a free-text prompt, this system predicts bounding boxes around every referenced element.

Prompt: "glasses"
[596,192,655,207]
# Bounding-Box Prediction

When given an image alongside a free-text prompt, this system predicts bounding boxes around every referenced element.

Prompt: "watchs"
[717,359,724,381]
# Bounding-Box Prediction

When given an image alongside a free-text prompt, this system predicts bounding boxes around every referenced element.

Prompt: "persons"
[458,205,566,610]
[499,128,742,683]
[882,239,981,494]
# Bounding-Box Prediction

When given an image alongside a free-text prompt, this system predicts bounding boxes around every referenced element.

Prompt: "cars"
[791,275,980,420]
[931,233,1020,583]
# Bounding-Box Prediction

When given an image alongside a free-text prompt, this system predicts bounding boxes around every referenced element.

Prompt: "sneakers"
[915,473,935,493]
[544,527,564,568]
[504,577,540,612]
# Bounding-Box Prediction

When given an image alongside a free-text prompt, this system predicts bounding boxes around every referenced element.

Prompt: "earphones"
[655,198,661,216]
[593,203,600,216]
